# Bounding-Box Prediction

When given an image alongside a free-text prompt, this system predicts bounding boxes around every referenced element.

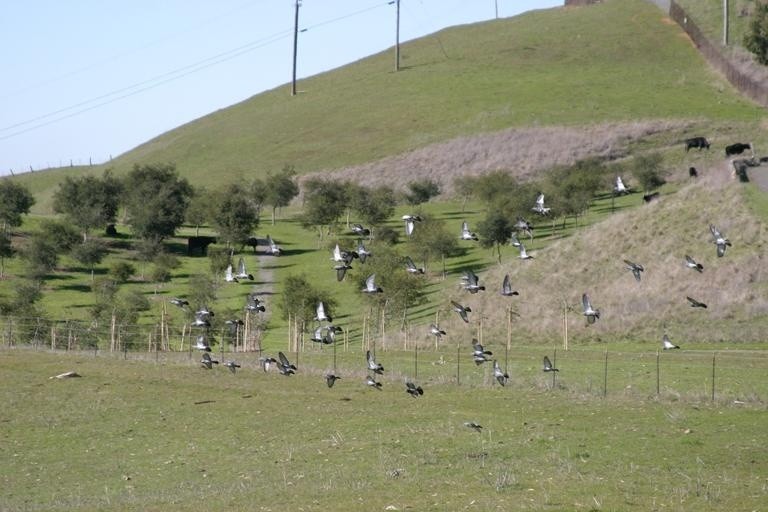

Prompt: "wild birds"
[685,223,733,273]
[580,294,600,325]
[170,212,535,398]
[541,354,560,374]
[533,193,552,218]
[622,257,644,282]
[612,177,632,195]
[661,332,678,350]
[686,295,708,311]
[463,419,485,435]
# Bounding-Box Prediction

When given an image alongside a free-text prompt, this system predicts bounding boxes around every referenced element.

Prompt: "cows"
[689,166,696,176]
[643,192,659,202]
[684,137,711,152]
[725,142,750,156]
